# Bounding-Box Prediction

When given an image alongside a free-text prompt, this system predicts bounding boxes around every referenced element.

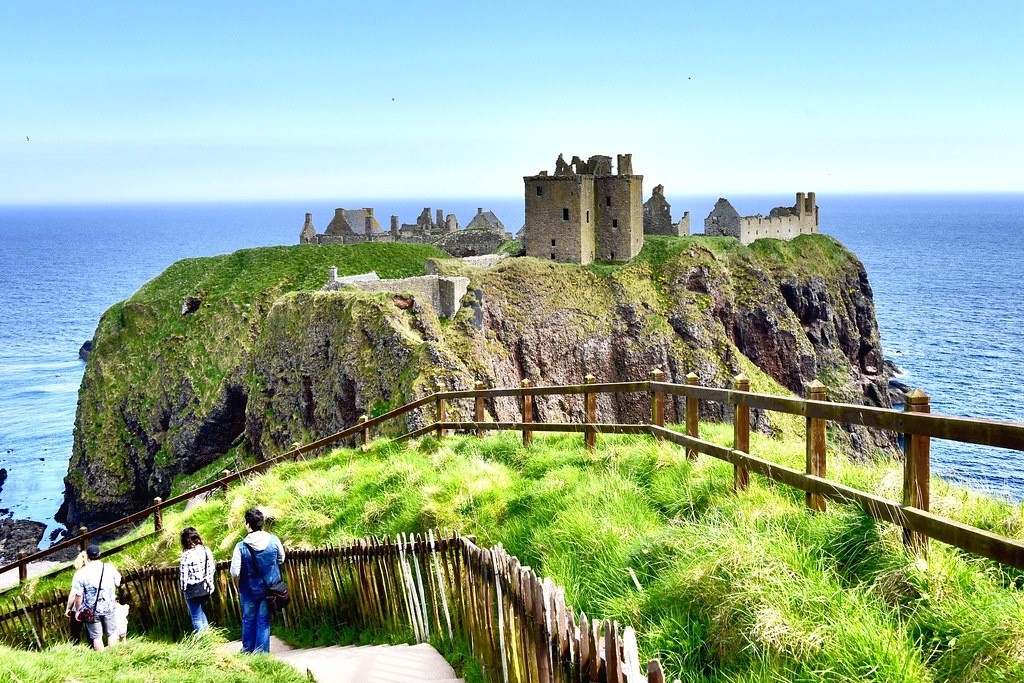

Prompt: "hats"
[87,545,101,558]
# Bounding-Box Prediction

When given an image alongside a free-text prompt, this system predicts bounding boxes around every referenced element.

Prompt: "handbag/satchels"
[266,582,290,613]
[78,609,94,623]
[187,580,211,603]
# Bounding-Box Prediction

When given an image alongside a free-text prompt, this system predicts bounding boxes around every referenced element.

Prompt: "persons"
[179,527,215,633]
[65,545,129,650]
[229,509,285,656]
